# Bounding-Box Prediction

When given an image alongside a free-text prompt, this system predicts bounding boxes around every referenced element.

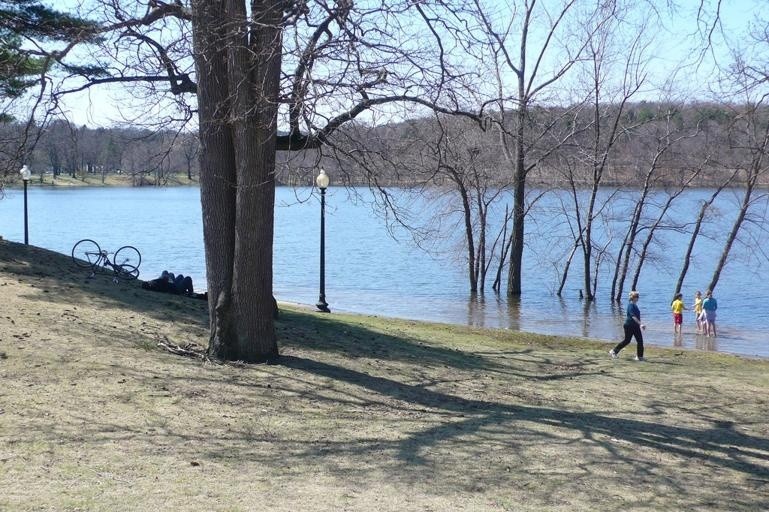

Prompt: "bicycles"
[70,237,141,288]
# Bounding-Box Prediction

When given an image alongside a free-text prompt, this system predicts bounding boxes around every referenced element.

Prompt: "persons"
[696,307,708,335]
[670,293,689,337]
[608,290,649,362]
[691,290,704,334]
[141,269,176,291]
[701,289,718,337]
[151,273,197,298]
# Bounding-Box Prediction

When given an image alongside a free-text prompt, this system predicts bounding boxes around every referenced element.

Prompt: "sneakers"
[610,349,618,358]
[636,356,647,361]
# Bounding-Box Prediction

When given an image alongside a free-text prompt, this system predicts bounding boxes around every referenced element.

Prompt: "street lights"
[314,167,334,314]
[18,163,33,246]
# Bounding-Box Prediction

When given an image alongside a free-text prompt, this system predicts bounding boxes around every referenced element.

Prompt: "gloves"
[640,325,646,329]
